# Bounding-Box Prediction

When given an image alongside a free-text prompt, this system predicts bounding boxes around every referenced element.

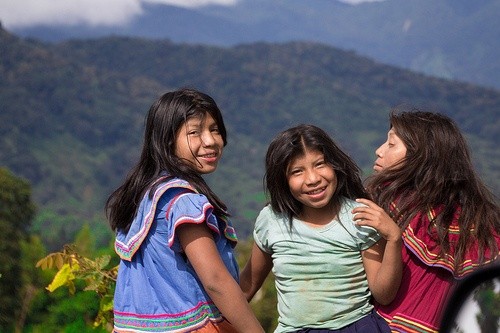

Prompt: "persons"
[365,109,500,333]
[238,124,403,333]
[105,88,267,333]
